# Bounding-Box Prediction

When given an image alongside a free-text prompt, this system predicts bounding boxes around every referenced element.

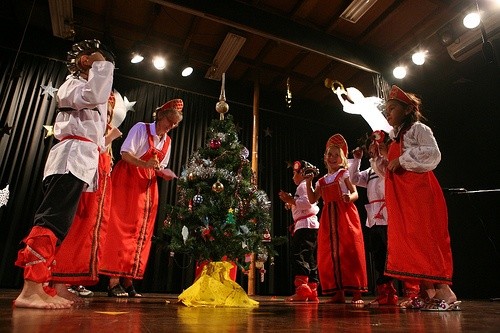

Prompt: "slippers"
[107,283,142,298]
[400,296,462,310]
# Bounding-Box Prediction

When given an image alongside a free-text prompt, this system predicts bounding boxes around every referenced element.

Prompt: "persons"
[13,37,128,308]
[369,85,463,311]
[279,131,388,305]
[93,99,184,297]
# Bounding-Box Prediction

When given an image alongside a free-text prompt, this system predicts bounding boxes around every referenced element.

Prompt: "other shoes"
[67,286,94,298]
